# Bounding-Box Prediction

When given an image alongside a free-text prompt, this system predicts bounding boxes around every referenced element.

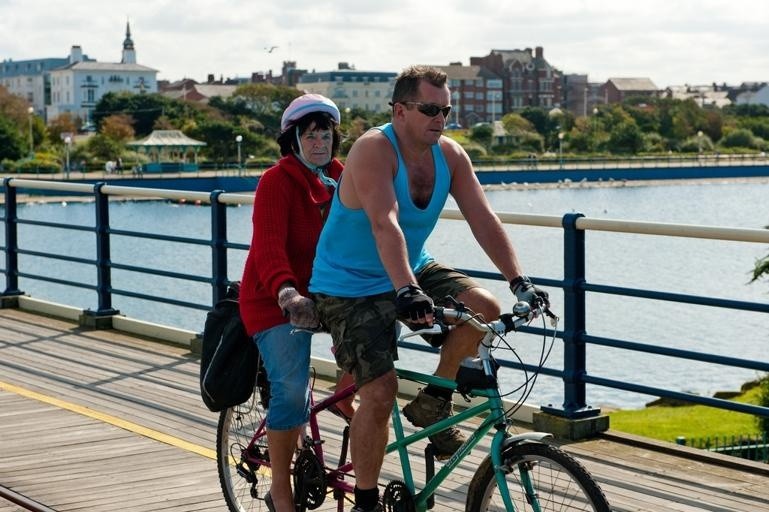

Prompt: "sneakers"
[351,503,386,512]
[265,492,276,512]
[403,389,471,458]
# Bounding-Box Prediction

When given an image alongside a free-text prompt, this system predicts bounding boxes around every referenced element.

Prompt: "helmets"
[280,95,341,130]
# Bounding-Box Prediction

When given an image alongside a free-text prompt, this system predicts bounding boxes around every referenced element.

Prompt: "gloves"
[276,288,318,330]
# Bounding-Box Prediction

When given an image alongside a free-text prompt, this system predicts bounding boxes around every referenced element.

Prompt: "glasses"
[389,101,452,119]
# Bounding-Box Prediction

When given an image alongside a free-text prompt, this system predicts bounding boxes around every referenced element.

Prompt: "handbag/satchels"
[199,281,270,413]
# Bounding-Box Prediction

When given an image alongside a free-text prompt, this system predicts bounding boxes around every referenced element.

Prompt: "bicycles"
[216,292,611,512]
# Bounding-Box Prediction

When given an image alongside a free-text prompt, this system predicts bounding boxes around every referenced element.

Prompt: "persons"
[65,157,144,179]
[236,92,345,511]
[305,66,549,511]
[527,153,537,170]
[169,148,196,163]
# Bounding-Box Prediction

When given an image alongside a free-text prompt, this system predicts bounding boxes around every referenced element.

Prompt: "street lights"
[559,133,564,167]
[64,137,71,178]
[236,136,243,175]
[27,106,35,154]
